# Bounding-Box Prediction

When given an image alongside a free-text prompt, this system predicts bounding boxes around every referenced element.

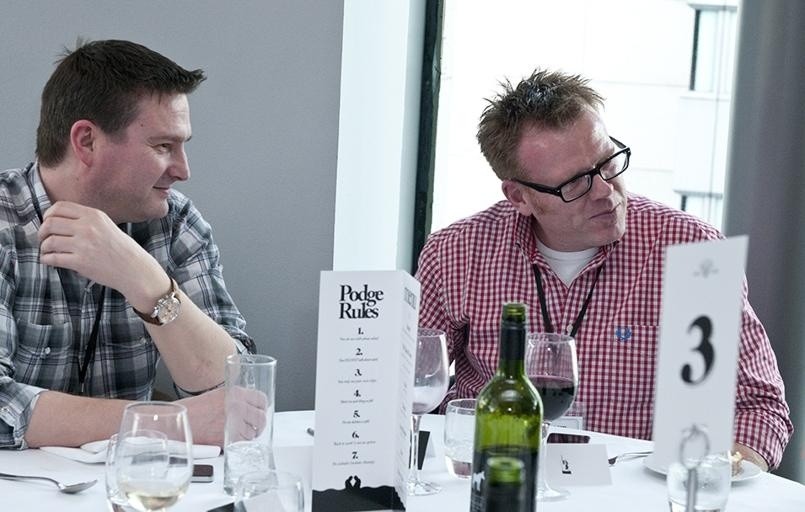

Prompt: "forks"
[606,452,656,465]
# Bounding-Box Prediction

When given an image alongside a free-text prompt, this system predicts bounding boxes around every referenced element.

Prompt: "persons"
[0,38,269,451]
[414,68,795,473]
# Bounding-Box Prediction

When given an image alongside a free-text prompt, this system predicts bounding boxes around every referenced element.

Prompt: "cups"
[231,468,305,512]
[218,350,278,498]
[113,400,194,511]
[667,458,733,512]
[105,427,170,512]
[438,396,485,484]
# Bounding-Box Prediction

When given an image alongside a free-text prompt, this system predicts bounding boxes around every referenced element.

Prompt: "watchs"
[133,277,181,327]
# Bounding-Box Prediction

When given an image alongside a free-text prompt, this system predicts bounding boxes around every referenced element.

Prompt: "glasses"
[509,136,631,205]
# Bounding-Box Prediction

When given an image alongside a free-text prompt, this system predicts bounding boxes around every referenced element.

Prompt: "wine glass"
[401,327,451,497]
[518,331,578,503]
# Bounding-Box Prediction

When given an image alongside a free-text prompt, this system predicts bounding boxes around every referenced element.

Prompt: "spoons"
[0,471,99,494]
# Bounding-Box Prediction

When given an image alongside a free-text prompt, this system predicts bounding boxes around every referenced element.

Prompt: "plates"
[642,450,763,486]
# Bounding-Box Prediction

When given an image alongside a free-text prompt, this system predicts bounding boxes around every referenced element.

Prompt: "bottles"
[467,301,542,512]
